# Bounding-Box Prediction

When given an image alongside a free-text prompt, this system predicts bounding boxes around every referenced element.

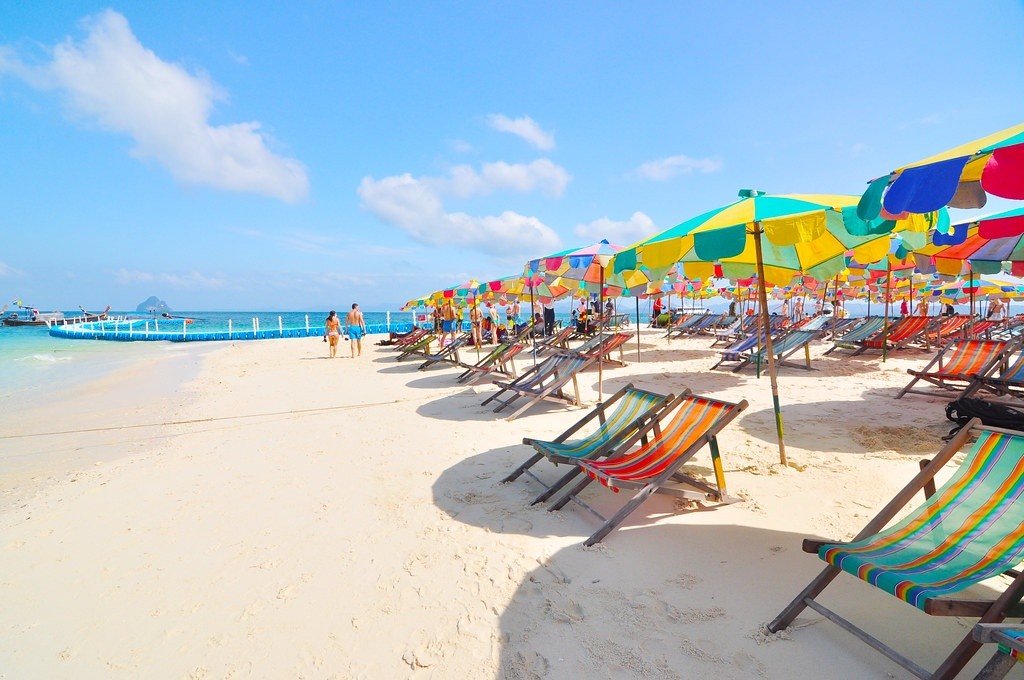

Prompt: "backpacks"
[945,397,1024,430]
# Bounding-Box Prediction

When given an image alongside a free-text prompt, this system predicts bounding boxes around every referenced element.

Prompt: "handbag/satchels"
[987,311,993,318]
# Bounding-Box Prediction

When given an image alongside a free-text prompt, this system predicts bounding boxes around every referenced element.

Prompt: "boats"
[79,305,174,321]
[2,305,110,327]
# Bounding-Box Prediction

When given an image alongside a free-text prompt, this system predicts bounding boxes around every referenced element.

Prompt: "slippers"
[942,427,963,440]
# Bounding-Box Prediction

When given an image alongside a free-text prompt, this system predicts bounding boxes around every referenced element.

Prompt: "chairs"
[393,311,1024,680]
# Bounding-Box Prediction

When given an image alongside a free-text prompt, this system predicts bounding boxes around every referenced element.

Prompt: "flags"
[13,301,21,306]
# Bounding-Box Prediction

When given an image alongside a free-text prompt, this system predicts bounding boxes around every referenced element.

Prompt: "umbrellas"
[400,124,1024,466]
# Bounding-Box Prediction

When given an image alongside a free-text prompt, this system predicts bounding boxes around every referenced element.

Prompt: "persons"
[319,298,1007,359]
